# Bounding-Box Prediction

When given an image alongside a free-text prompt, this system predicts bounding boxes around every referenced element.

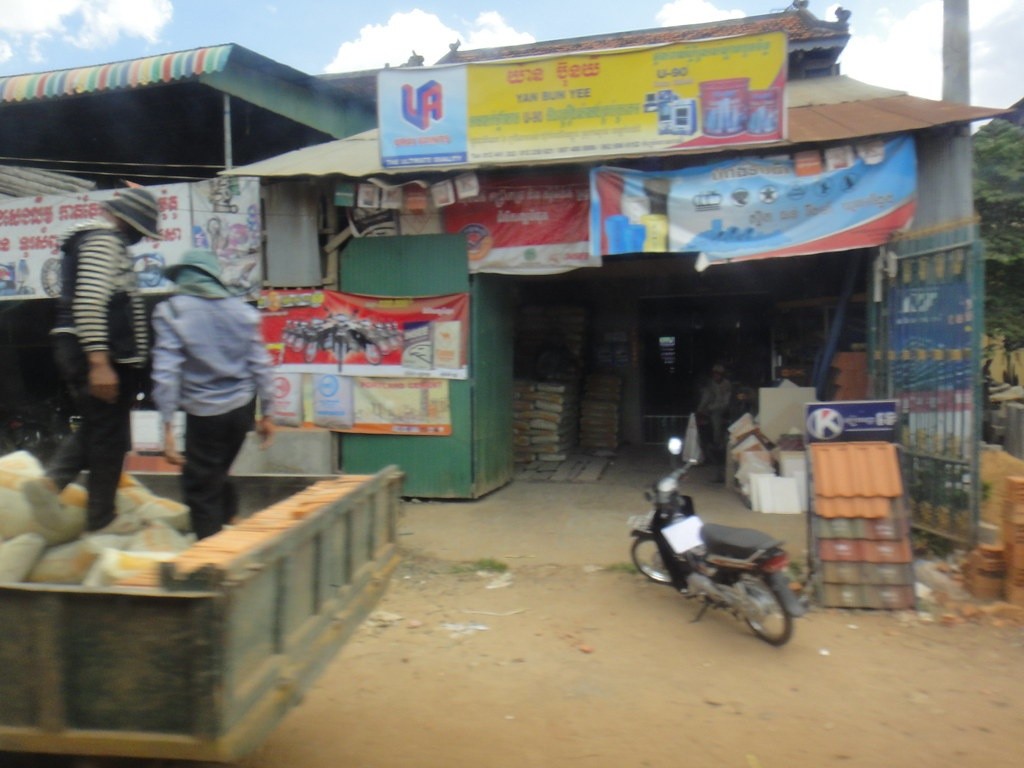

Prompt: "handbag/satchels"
[681,413,707,465]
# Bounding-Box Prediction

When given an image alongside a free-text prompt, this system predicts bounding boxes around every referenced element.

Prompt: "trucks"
[0,460,412,768]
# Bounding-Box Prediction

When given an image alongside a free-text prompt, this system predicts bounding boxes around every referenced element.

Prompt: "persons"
[44,185,161,536]
[148,250,277,542]
[695,365,732,450]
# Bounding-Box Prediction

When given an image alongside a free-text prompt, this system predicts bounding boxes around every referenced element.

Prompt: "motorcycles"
[632,438,802,648]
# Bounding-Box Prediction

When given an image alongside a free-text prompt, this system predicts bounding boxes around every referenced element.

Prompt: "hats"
[163,248,237,300]
[100,187,163,241]
